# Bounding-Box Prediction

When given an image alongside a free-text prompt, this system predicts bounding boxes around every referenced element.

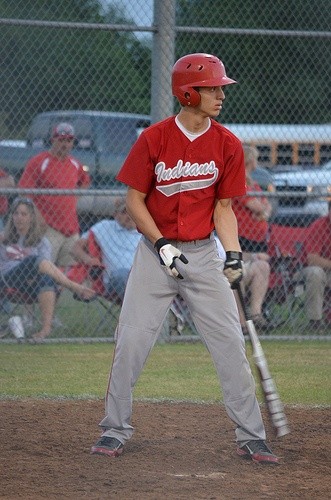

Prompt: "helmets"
[168,53,237,108]
[48,123,79,147]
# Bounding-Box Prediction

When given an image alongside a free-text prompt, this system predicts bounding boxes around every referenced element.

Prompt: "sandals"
[243,312,274,335]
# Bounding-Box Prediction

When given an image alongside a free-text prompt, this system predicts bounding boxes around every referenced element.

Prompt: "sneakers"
[236,435,277,465]
[88,436,124,457]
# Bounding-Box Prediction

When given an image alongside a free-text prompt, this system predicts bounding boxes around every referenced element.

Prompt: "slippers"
[72,287,102,305]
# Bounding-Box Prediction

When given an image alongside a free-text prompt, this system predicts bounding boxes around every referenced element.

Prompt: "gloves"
[152,238,190,283]
[223,250,247,288]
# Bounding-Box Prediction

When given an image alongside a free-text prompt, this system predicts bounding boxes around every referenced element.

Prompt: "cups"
[9,316,24,339]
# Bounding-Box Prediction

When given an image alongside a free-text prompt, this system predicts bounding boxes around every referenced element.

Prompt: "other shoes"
[311,319,326,334]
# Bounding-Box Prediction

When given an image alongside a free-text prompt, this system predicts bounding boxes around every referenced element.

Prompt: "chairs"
[1,230,125,339]
[267,225,310,330]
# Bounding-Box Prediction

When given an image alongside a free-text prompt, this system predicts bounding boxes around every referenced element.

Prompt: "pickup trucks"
[0,110,331,230]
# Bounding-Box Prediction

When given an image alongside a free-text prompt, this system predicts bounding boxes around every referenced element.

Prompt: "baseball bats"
[234,281,293,443]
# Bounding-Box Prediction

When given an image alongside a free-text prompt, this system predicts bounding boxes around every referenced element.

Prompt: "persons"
[90,53,279,464]
[0,122,273,336]
[293,201,331,333]
